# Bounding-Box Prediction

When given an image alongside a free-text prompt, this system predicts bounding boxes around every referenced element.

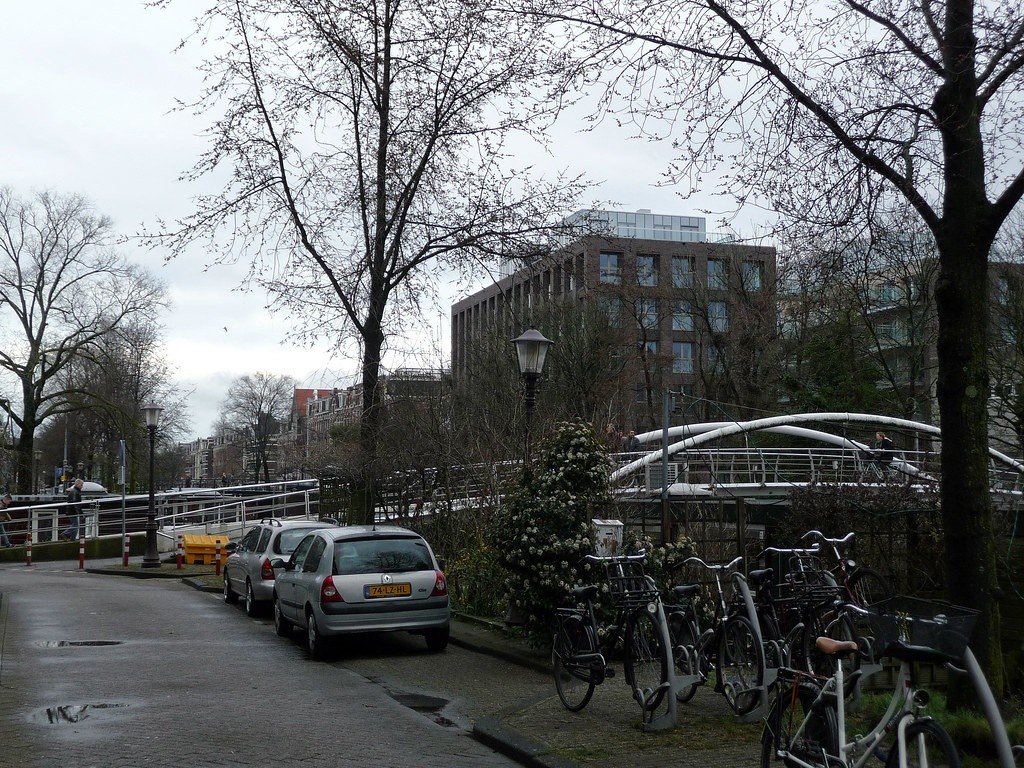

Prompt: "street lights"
[62,460,85,492]
[509,325,555,488]
[33,449,42,495]
[139,400,165,569]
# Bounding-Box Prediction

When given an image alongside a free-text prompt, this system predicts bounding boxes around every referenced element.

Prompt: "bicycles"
[757,593,985,768]
[751,543,861,703]
[857,447,903,488]
[552,548,671,713]
[615,457,643,488]
[799,529,890,631]
[658,556,765,716]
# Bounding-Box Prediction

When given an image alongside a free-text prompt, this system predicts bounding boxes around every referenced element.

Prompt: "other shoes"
[58,533,70,543]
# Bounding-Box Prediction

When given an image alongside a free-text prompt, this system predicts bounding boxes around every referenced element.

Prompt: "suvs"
[224,517,339,617]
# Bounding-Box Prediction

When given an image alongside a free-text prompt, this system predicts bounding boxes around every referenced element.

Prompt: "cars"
[273,526,451,663]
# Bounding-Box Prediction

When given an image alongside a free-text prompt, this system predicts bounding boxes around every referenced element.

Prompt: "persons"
[60,479,85,541]
[607,426,640,487]
[222,473,227,487]
[873,432,895,482]
[0,495,14,548]
[186,475,192,488]
